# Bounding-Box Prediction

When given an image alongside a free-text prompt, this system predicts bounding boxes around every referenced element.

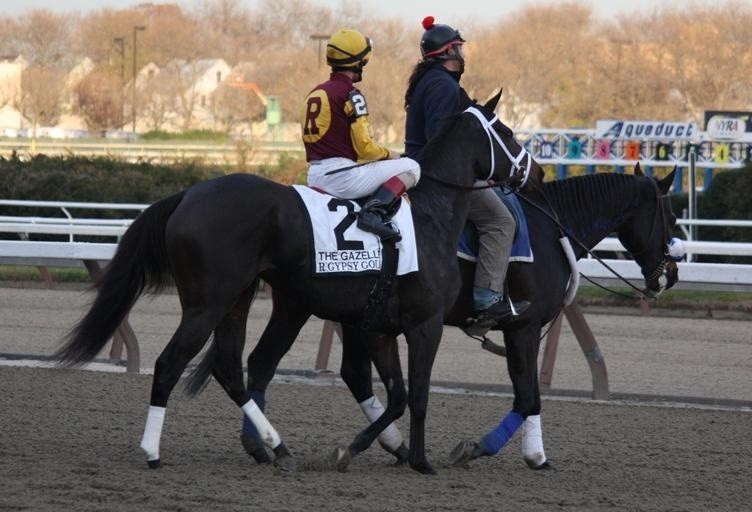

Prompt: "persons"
[404,16,532,336]
[300,28,422,240]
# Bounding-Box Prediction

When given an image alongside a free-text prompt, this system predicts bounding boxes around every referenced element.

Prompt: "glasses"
[447,29,465,54]
[355,35,373,63]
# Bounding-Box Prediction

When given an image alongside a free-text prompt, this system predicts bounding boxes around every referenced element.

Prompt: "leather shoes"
[475,301,531,321]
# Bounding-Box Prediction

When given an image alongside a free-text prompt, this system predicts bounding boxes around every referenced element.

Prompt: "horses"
[178,162,679,471]
[37,87,546,475]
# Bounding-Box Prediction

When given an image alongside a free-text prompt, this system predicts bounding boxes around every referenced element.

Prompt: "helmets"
[420,16,466,57]
[326,29,371,66]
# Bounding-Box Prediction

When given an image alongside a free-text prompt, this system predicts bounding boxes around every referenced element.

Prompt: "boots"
[357,176,406,242]
